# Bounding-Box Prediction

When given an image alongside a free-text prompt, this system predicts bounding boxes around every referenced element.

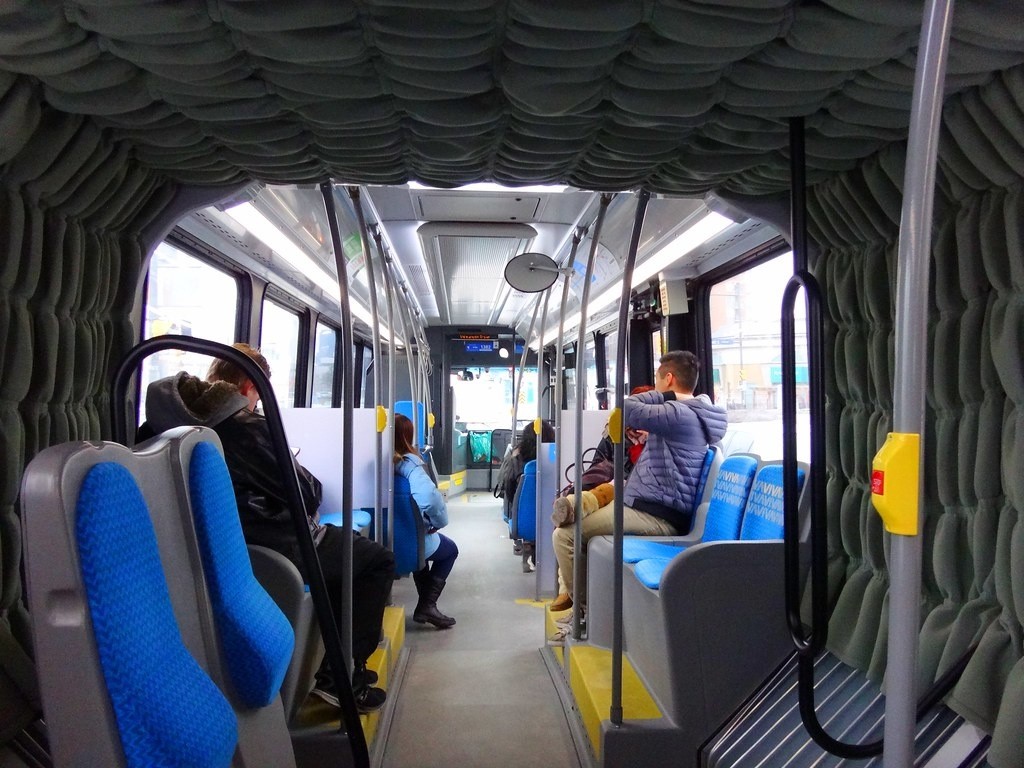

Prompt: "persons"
[499,420,556,573]
[138,343,396,714]
[545,350,728,647]
[394,413,459,629]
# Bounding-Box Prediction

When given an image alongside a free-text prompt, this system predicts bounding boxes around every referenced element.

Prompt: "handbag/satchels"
[494,476,518,503]
[565,448,614,494]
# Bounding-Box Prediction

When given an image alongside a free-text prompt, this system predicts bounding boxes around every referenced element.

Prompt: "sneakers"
[548,624,573,646]
[555,607,586,628]
[315,658,379,688]
[310,679,387,713]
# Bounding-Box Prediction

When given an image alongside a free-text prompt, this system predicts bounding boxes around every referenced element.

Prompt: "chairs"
[275,401,830,731]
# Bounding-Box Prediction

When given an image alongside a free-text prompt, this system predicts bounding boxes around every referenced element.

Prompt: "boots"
[413,573,456,627]
[413,565,430,594]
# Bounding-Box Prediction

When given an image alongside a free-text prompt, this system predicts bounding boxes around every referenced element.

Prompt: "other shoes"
[527,556,535,570]
[551,496,573,528]
[549,594,573,611]
[514,539,522,554]
